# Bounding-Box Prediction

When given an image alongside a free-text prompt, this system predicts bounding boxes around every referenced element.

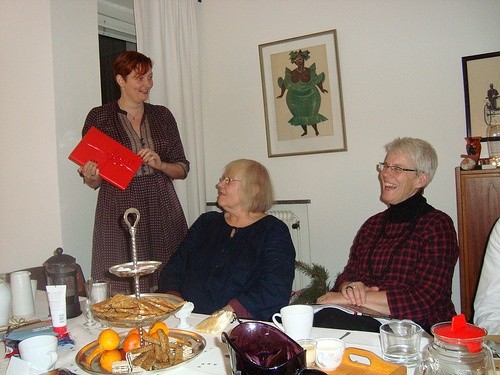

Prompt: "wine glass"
[174,302,194,330]
[78,299,111,330]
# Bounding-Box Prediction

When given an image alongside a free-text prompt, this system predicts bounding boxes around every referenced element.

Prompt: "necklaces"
[366,209,422,279]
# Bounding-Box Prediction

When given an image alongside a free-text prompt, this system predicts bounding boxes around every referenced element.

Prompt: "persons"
[305,136,460,337]
[473,217,500,336]
[156,159,296,324]
[77,50,191,306]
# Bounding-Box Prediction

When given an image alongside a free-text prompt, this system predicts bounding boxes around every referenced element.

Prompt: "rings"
[345,286,353,292]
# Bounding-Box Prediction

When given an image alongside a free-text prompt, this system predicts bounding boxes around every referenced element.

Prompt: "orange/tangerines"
[98,321,168,353]
[100,349,122,371]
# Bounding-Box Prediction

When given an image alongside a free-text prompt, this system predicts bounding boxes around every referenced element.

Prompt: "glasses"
[378,161,424,174]
[219,176,240,185]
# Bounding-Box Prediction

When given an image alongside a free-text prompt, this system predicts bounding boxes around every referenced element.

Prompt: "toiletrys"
[45,284,68,335]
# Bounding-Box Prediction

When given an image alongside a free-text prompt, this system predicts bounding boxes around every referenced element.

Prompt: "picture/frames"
[462,51,500,142]
[259,29,348,158]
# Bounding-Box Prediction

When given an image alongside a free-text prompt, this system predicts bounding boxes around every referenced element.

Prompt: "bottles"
[43,247,83,319]
[465,137,482,163]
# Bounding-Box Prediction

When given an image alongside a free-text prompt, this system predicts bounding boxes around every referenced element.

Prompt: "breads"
[195,316,218,332]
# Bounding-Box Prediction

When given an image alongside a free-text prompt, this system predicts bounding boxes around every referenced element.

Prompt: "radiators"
[266,211,304,291]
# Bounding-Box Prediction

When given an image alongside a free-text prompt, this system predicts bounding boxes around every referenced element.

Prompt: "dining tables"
[0,301,433,375]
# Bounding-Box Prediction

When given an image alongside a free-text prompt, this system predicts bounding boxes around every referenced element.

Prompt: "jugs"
[421,315,496,374]
[220,322,328,375]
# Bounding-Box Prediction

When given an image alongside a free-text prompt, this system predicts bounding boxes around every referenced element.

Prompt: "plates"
[484,335,500,368]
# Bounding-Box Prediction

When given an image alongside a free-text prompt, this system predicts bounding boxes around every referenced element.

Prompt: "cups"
[271,305,314,341]
[0,271,37,329]
[18,335,59,372]
[296,339,317,367]
[379,321,421,368]
[86,280,107,305]
[314,338,345,371]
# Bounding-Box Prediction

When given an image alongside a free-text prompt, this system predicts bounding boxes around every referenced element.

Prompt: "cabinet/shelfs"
[456,167,500,325]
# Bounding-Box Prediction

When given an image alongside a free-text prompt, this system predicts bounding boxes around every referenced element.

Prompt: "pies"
[92,294,187,327]
